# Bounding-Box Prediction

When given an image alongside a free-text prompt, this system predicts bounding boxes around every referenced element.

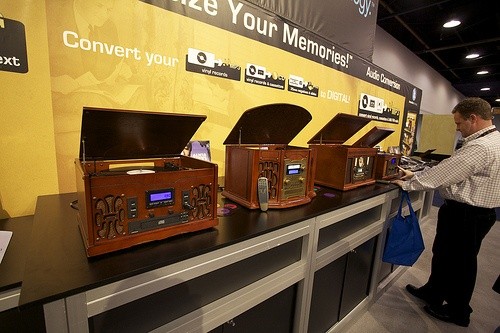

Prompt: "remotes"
[258,177,269,211]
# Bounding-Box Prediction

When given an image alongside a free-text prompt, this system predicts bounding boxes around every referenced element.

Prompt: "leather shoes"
[406,284,449,305]
[424,304,470,327]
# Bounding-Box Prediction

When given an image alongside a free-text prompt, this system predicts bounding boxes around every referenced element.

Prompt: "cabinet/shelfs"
[0,175,435,333]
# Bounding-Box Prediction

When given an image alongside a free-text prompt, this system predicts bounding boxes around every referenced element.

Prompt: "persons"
[390,97,500,327]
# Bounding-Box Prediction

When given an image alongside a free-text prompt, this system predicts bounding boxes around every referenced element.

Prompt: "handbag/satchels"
[382,190,425,267]
